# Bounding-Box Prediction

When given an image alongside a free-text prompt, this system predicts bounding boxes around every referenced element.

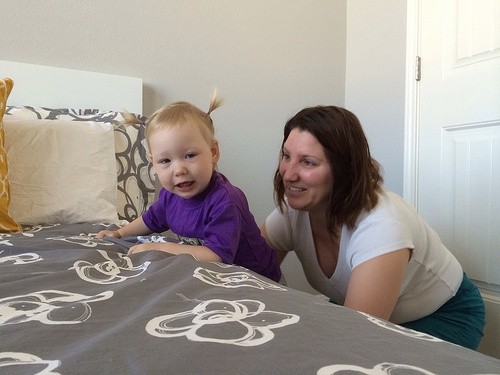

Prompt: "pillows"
[0,77,23,231]
[3,118,121,232]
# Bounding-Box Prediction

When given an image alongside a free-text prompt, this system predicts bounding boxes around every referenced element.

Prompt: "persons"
[259,105,486,351]
[95,87,287,287]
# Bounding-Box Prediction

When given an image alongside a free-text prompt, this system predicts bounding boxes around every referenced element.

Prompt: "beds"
[0,59,499,375]
